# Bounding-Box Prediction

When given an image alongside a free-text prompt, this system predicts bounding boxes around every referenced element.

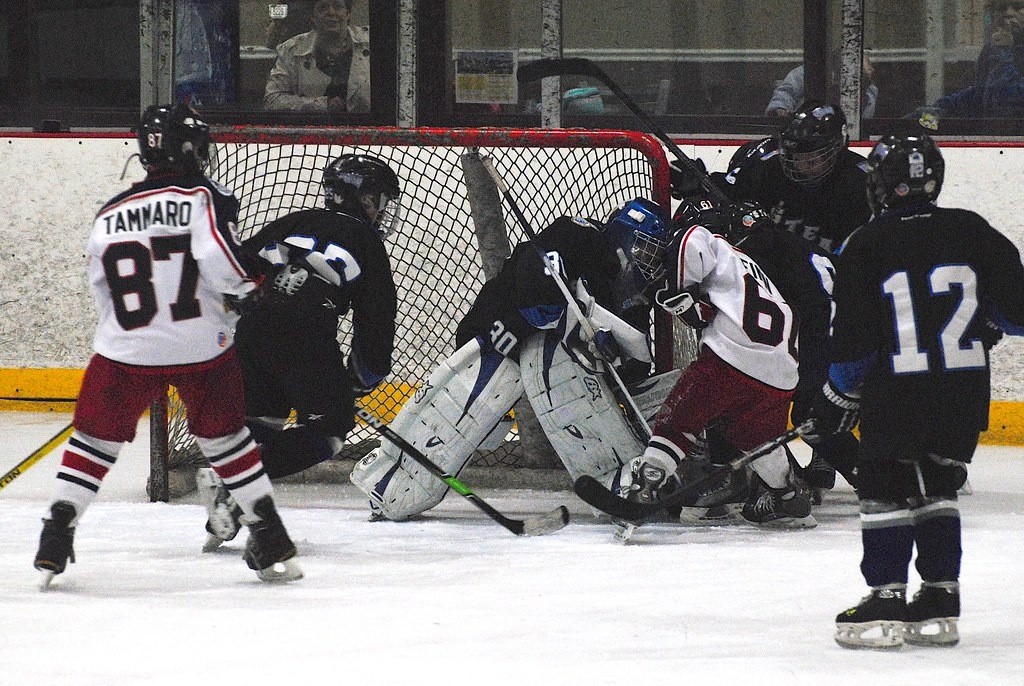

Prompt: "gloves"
[668,157,709,203]
[720,200,768,237]
[346,358,380,397]
[793,376,862,446]
[655,280,719,330]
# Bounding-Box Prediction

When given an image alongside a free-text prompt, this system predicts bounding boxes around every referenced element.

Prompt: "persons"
[198,154,401,541]
[808,128,1024,622]
[264,0,371,123]
[617,220,813,526]
[763,0,1024,117]
[351,197,673,521]
[34,102,296,575]
[669,97,875,508]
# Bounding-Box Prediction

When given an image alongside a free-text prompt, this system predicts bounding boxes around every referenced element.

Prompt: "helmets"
[781,102,847,189]
[138,102,217,177]
[665,199,724,248]
[868,130,945,208]
[605,196,672,281]
[321,153,400,242]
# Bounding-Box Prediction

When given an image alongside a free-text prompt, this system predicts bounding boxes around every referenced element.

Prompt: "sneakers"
[33,501,76,590]
[835,584,906,650]
[196,469,245,552]
[242,497,305,582]
[905,581,961,646]
[589,453,835,543]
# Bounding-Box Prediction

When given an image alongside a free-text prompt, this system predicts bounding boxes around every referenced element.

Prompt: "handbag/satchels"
[560,88,604,115]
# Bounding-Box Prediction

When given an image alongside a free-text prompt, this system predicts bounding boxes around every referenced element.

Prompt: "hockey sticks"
[478,153,746,507]
[575,417,817,525]
[354,405,571,537]
[0,421,76,489]
[514,56,734,206]
